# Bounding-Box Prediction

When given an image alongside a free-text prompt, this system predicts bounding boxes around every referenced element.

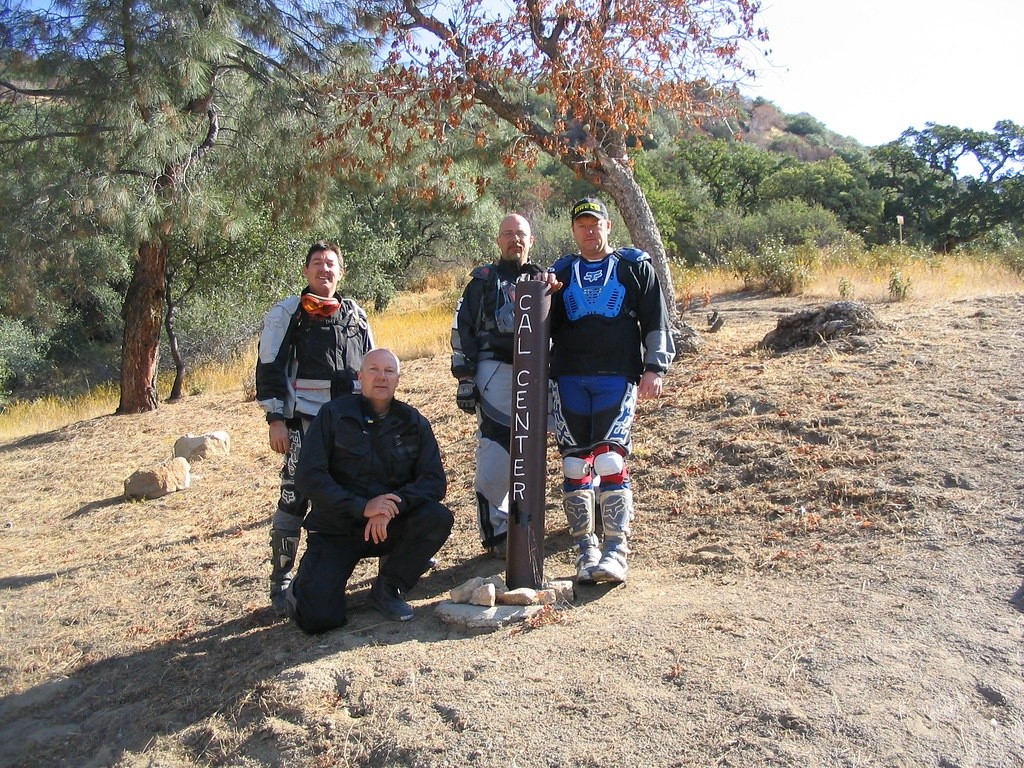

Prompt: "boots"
[562,488,602,582]
[268,529,301,617]
[592,488,634,582]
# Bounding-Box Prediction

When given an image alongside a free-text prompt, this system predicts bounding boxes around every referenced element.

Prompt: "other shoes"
[484,538,507,558]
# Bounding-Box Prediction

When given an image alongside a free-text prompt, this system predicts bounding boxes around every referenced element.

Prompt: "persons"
[257,241,377,618]
[535,198,676,585]
[286,348,455,635]
[451,214,550,562]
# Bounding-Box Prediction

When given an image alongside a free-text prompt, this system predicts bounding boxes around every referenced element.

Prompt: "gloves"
[456,376,482,416]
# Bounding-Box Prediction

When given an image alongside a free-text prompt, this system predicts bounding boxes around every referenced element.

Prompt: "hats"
[570,197,608,221]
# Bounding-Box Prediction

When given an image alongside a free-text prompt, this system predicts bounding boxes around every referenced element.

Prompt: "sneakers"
[367,583,414,621]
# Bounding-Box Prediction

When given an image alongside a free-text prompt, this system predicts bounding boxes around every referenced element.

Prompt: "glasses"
[301,292,341,316]
[499,230,527,238]
